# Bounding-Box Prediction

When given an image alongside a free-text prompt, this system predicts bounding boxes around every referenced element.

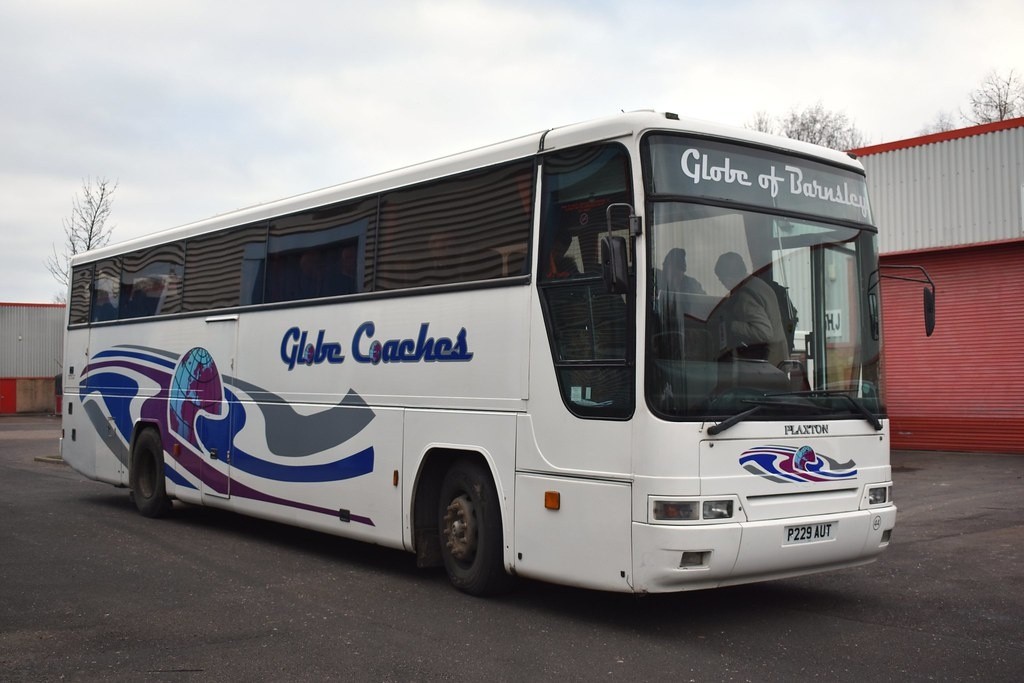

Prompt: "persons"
[712,251,791,393]
[539,226,578,278]
[654,247,710,295]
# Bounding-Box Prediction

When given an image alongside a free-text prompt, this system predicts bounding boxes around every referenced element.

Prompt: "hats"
[663,248,687,272]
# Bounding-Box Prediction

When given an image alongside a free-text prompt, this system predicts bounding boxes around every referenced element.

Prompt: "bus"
[59,110,936,599]
